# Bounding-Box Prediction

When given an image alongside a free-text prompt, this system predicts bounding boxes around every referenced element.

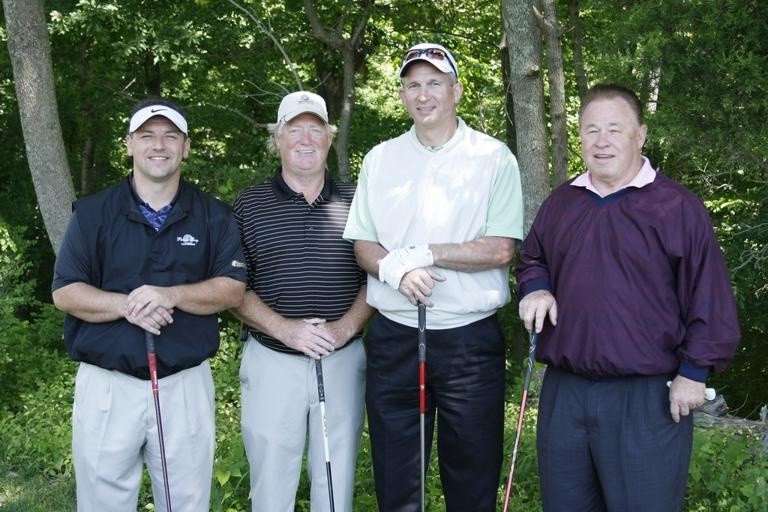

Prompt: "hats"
[400,43,458,79]
[277,91,328,124]
[130,100,188,136]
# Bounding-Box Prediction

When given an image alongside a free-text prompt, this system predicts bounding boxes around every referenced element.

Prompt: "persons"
[50,100,247,512]
[512,81,743,512]
[228,90,376,512]
[343,41,527,510]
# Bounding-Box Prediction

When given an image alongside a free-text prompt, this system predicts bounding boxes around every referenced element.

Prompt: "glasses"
[403,47,457,78]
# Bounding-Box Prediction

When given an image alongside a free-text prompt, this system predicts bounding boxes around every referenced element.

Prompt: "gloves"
[378,242,434,289]
